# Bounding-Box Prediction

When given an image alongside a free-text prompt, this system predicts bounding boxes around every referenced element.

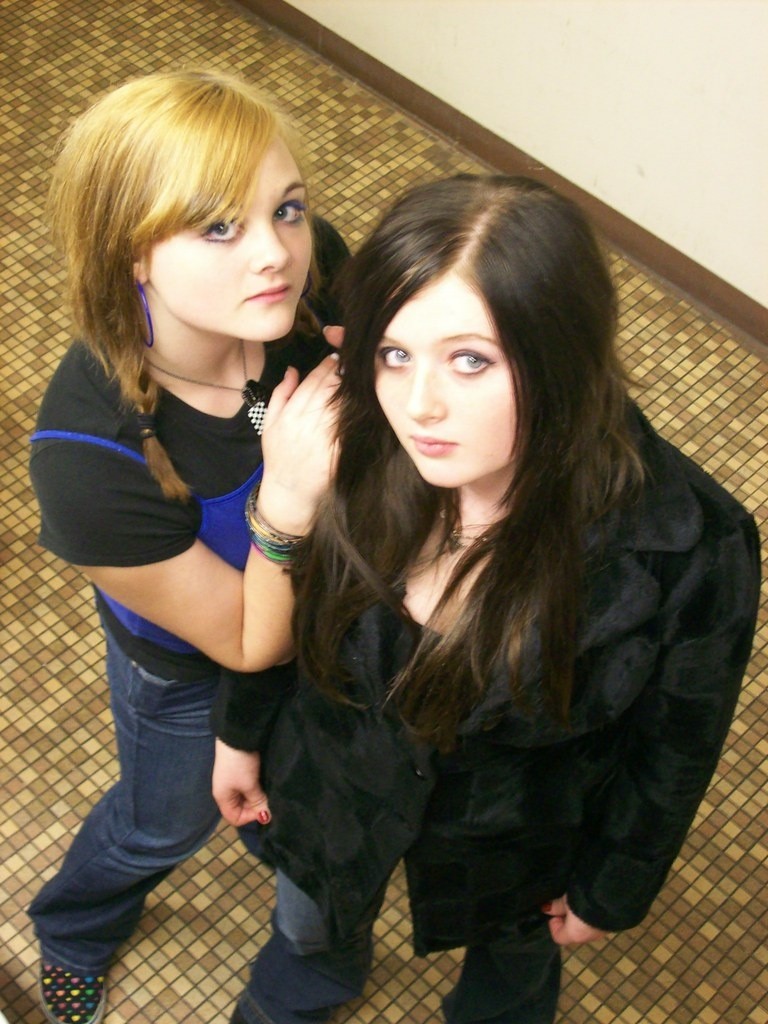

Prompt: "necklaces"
[440,508,490,542]
[145,338,265,438]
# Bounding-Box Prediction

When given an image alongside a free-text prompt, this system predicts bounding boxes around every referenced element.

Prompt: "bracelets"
[244,480,307,565]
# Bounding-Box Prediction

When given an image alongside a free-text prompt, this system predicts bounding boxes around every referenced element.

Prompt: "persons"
[210,165,760,1024]
[26,62,357,1024]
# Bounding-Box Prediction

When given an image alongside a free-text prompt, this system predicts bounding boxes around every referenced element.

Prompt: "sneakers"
[39,952,106,1024]
